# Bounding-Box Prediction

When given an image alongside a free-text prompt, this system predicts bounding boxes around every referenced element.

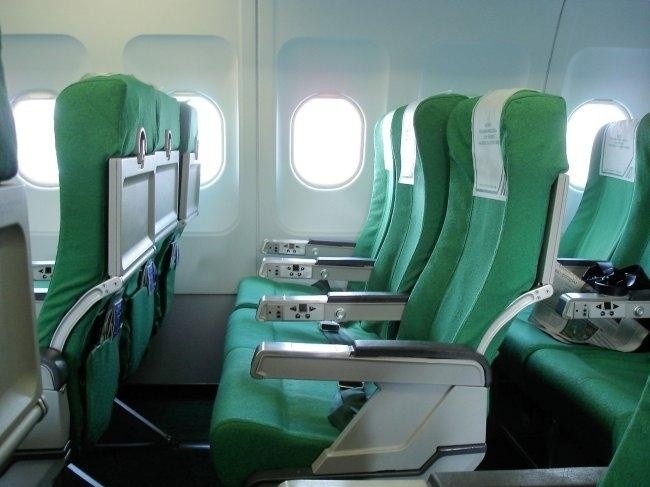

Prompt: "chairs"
[221,90,470,367]
[119,90,182,381]
[280,374,650,487]
[207,88,570,487]
[524,112,650,464]
[18,73,157,452]
[0,27,104,487]
[501,117,642,367]
[152,102,200,332]
[231,103,409,312]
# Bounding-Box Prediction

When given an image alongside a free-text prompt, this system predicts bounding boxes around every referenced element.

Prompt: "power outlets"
[568,300,590,320]
[260,258,281,280]
[255,295,283,321]
[262,239,280,255]
